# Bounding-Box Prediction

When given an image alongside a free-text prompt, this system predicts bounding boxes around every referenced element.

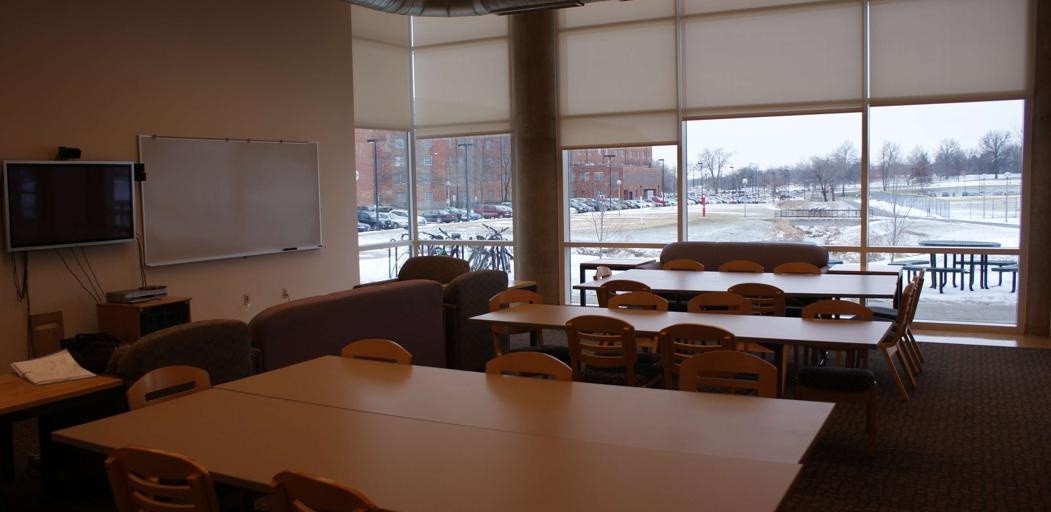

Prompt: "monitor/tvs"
[3,159,137,253]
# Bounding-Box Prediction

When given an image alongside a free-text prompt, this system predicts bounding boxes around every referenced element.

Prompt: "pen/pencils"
[281,247,301,252]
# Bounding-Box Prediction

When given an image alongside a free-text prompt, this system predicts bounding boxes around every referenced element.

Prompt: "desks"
[579,256,655,307]
[918,239,999,293]
[0,373,123,511]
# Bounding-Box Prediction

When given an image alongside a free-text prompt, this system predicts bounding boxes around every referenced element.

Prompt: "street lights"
[456,143,473,221]
[742,179,748,217]
[604,155,617,211]
[698,163,704,205]
[366,137,379,230]
[617,180,622,216]
[659,159,665,207]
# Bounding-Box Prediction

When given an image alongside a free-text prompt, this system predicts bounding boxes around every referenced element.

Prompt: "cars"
[358,202,513,232]
[570,191,766,214]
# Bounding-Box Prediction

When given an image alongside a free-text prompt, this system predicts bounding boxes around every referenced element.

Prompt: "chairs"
[662,258,821,277]
[835,270,924,400]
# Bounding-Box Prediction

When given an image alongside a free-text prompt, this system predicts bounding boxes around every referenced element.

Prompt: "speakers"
[61,332,123,374]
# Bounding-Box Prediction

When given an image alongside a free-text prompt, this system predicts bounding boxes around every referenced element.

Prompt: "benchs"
[992,266,1019,295]
[906,266,968,294]
[890,259,927,279]
[955,258,1014,289]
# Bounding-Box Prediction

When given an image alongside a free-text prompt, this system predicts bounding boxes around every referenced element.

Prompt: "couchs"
[353,256,470,294]
[636,242,829,271]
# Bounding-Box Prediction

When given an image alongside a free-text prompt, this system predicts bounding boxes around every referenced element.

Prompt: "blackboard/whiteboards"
[139,135,324,267]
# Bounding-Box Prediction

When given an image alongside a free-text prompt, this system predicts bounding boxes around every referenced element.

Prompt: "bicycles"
[390,222,513,274]
